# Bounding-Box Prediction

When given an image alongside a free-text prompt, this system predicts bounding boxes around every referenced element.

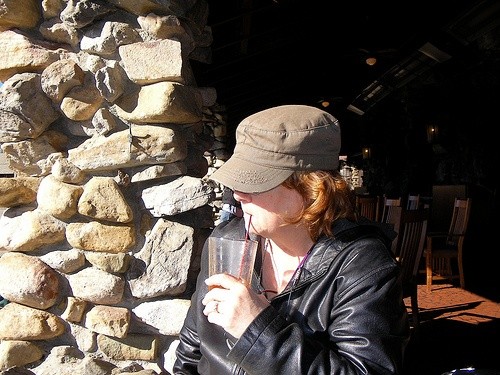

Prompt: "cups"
[208,237,258,291]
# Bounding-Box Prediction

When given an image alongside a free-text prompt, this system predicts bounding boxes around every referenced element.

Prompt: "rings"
[214,303,219,313]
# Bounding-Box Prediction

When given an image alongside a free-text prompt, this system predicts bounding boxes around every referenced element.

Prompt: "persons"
[174,105,404,375]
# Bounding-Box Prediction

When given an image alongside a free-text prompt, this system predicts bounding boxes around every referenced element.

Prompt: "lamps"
[366,51,377,66]
[361,143,372,159]
[427,124,439,143]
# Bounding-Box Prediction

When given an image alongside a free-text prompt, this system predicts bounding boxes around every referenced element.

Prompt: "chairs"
[356,185,470,365]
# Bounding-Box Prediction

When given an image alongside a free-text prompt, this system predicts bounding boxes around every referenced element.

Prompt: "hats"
[207,105,342,194]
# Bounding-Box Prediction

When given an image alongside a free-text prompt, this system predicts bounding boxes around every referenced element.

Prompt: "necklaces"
[259,238,315,302]
